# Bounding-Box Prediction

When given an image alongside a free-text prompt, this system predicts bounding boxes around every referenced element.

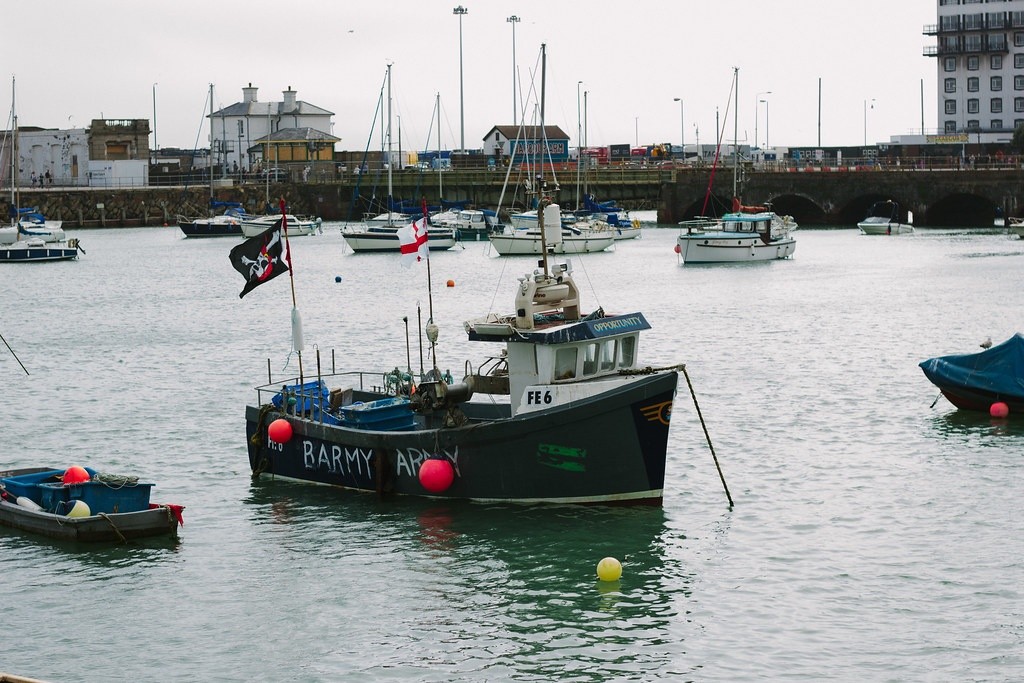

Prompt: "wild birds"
[348,29,354,33]
[980,336,993,351]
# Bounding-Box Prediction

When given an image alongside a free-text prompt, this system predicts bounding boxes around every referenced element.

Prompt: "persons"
[257,167,266,183]
[956,150,1018,170]
[302,164,310,183]
[242,166,247,184]
[30,169,55,189]
[233,160,238,173]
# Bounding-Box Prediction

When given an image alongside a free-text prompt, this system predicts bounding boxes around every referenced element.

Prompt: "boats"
[245,196,687,508]
[0,467,186,535]
[1009,217,1024,240]
[918,332,1024,420]
[857,199,915,235]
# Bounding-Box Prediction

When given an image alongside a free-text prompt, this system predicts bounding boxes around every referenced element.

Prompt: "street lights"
[507,15,522,167]
[153,83,159,164]
[755,91,773,148]
[452,5,469,168]
[674,98,685,164]
[865,98,875,154]
[761,99,768,148]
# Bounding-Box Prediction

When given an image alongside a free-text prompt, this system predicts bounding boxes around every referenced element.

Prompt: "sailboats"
[677,67,799,264]
[362,114,413,228]
[340,65,641,254]
[177,83,243,237]
[0,76,66,244]
[0,115,86,262]
[239,101,322,238]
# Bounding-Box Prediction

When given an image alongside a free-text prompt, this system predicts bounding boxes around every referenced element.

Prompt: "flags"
[228,218,292,300]
[396,217,429,262]
